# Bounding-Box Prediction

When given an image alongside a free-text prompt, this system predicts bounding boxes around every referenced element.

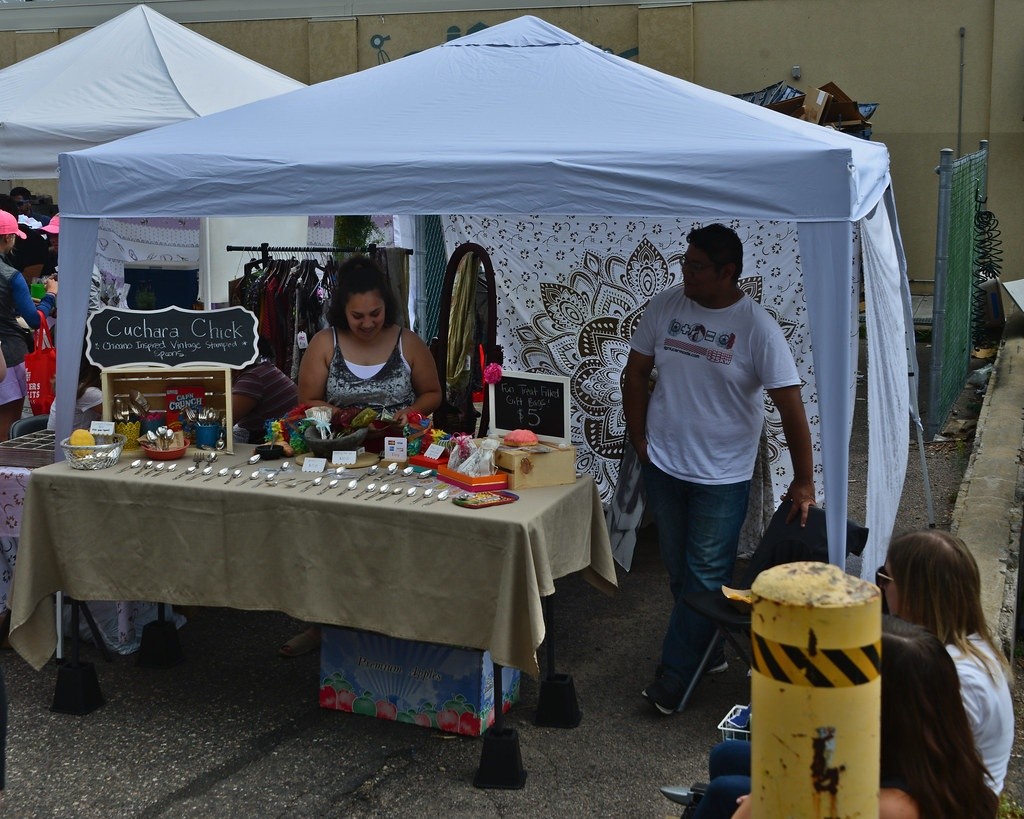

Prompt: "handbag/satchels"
[25,309,57,416]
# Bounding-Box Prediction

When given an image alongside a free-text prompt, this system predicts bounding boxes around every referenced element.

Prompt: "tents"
[36,11,932,658]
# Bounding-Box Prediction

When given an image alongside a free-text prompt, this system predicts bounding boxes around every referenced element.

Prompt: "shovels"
[126,388,152,419]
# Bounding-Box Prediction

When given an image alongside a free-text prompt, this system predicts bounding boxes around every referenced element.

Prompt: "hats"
[0,209,27,241]
[41,213,61,235]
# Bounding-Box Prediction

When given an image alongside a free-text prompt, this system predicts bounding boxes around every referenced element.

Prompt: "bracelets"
[45,291,57,297]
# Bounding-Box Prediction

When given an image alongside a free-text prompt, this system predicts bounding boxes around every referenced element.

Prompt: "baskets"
[60,433,127,470]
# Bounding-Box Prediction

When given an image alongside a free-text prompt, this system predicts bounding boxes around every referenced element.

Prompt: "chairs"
[673,500,870,714]
[10,414,49,440]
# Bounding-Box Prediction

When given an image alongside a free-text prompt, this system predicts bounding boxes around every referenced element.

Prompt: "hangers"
[235,256,338,298]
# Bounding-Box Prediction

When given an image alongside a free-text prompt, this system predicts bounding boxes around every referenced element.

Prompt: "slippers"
[281,633,321,656]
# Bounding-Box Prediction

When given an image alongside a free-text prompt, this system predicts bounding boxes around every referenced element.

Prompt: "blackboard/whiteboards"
[489,370,571,447]
[84,305,260,370]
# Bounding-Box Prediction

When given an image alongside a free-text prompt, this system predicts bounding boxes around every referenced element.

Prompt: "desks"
[8,442,621,788]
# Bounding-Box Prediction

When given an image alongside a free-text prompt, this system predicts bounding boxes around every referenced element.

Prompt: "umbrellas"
[0,5,318,182]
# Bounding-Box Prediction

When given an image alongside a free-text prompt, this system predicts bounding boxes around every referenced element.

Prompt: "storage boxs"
[319,621,521,737]
[472,438,577,490]
[124,260,199,311]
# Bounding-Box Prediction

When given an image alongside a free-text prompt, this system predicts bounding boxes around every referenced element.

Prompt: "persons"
[283,253,436,657]
[40,211,59,269]
[41,347,106,440]
[11,200,47,240]
[0,342,7,382]
[726,614,1000,819]
[0,193,56,293]
[215,348,300,446]
[621,221,818,723]
[661,525,1015,819]
[0,209,58,442]
[9,187,52,229]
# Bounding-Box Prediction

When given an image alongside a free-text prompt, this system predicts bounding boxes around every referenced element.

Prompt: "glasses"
[15,200,29,206]
[875,566,895,589]
[680,256,720,271]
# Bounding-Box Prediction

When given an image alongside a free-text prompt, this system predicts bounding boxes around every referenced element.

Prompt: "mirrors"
[431,242,504,438]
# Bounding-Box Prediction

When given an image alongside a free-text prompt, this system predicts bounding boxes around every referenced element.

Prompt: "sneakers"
[704,659,728,674]
[640,666,684,714]
